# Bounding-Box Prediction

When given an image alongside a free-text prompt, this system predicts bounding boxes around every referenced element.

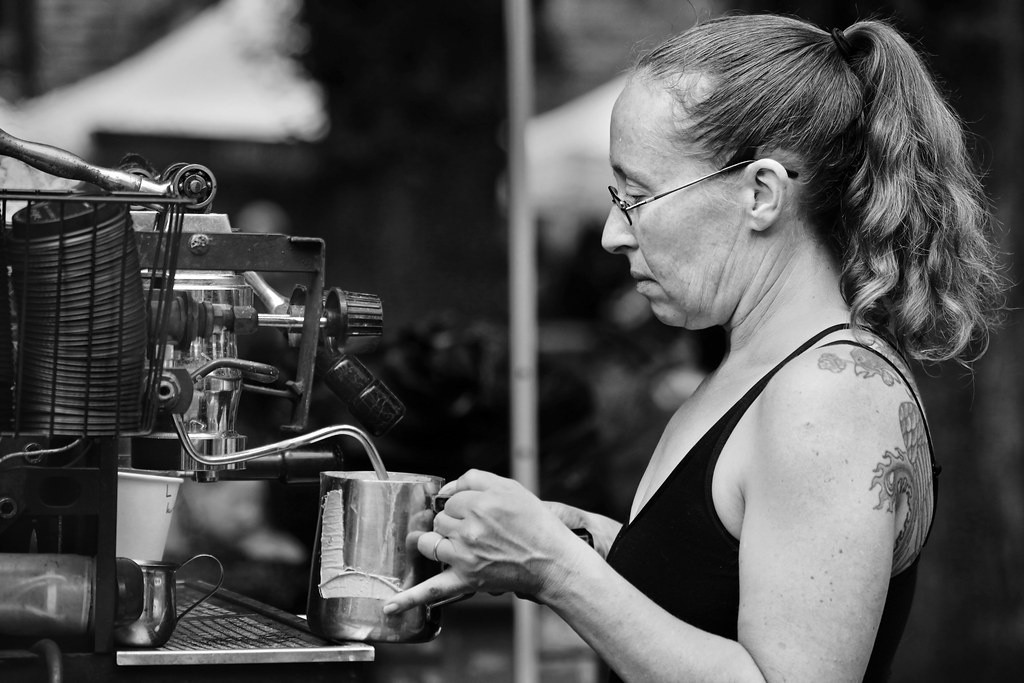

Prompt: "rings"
[434,537,447,561]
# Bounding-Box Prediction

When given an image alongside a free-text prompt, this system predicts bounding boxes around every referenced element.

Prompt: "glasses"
[605,158,800,227]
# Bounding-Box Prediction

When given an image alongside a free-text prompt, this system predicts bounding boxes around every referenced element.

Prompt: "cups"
[116,553,224,649]
[306,471,476,645]
[116,471,185,562]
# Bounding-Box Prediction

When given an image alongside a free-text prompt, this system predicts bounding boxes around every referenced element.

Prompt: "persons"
[382,14,1006,683]
